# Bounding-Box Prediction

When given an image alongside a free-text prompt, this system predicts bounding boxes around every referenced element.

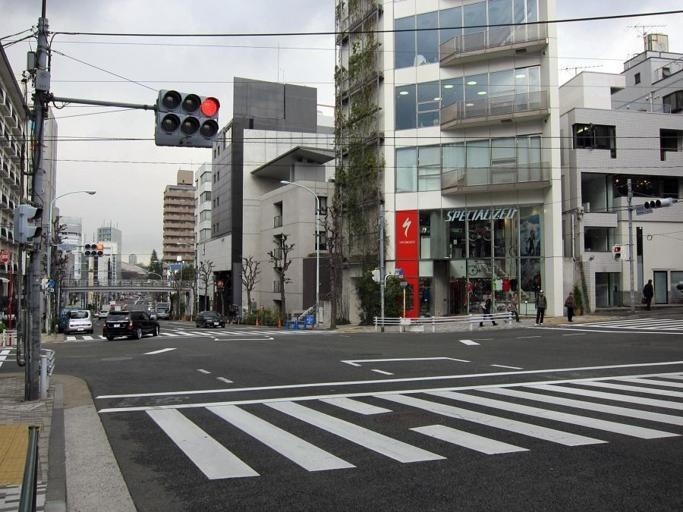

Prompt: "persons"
[535,290,547,326]
[565,292,576,322]
[526,230,535,256]
[504,292,520,323]
[479,295,498,326]
[643,279,653,311]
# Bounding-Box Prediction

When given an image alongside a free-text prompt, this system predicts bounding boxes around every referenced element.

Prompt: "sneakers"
[534,323,544,326]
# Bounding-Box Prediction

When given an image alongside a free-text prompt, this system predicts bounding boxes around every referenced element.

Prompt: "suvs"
[100,310,163,340]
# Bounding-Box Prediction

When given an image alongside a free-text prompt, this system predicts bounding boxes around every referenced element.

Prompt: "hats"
[539,290,544,294]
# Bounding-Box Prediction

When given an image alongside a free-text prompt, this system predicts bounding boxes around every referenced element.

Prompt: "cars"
[60,290,173,326]
[61,310,93,334]
[193,310,227,328]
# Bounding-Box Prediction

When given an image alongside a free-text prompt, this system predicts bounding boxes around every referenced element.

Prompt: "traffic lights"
[84,250,104,257]
[613,246,626,261]
[151,112,219,149]
[641,196,675,209]
[155,88,223,120]
[15,203,46,246]
[84,244,104,250]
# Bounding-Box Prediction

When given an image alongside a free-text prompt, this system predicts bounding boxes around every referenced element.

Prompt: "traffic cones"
[254,317,312,330]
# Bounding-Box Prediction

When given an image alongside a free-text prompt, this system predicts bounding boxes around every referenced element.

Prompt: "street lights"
[174,242,199,313]
[278,178,325,329]
[399,277,410,338]
[146,271,164,303]
[153,260,173,314]
[44,188,99,333]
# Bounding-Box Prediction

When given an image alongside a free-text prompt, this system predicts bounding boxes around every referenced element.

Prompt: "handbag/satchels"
[642,297,648,304]
